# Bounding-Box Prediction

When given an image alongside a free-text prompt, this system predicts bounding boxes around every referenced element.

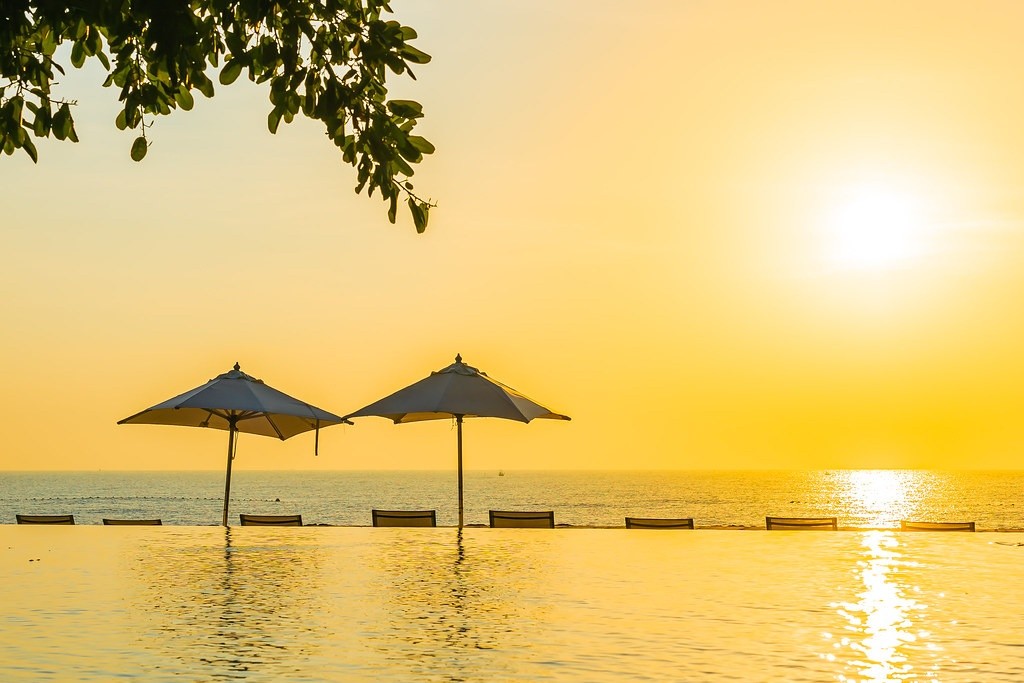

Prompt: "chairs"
[372,509,436,527]
[489,510,555,528]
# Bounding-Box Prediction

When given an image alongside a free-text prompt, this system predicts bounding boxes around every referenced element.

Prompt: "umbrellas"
[340,353,572,528]
[117,361,353,527]
